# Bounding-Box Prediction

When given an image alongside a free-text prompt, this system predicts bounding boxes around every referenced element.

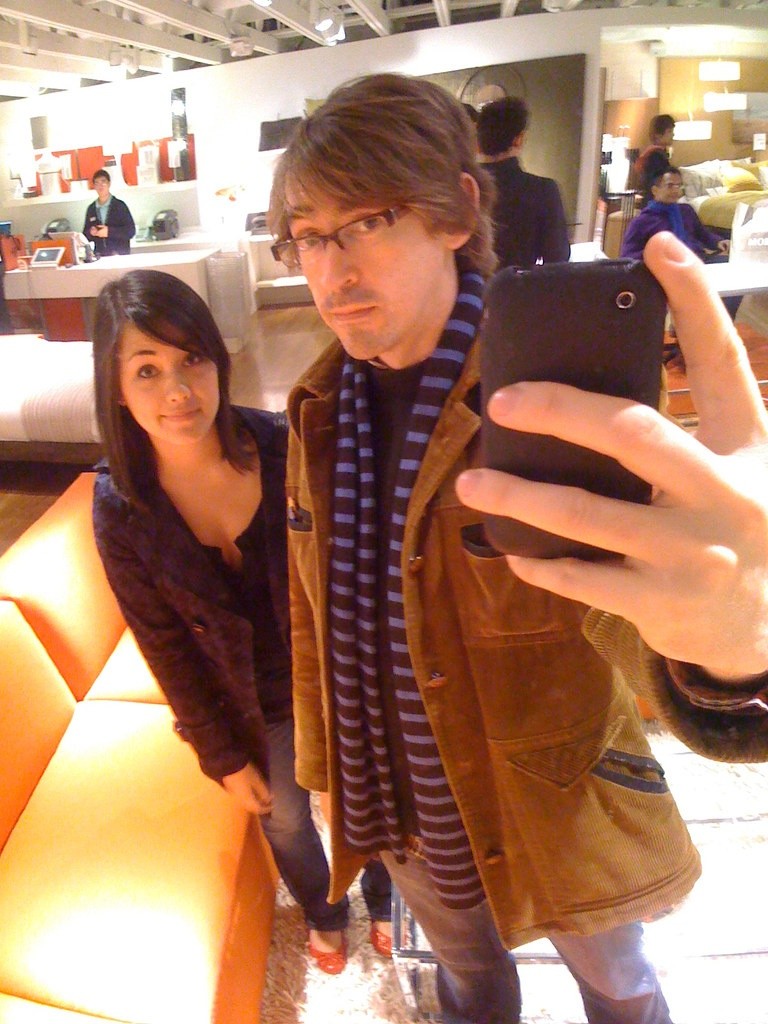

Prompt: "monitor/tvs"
[30,246,66,268]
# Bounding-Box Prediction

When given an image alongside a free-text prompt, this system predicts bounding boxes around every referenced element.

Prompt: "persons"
[631,112,678,207]
[92,269,394,976]
[266,71,768,1024]
[80,169,136,260]
[620,165,732,266]
[462,93,569,271]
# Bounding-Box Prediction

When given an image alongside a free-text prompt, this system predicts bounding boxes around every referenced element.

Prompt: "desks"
[665,261,768,396]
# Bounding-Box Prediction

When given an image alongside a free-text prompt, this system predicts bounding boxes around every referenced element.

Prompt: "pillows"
[678,158,767,198]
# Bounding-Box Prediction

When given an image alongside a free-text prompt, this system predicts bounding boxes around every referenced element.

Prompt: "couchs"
[0,463,279,1024]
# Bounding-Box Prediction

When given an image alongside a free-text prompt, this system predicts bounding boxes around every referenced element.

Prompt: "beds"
[679,192,767,262]
[0,332,102,467]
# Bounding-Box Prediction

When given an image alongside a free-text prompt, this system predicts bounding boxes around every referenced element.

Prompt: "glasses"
[271,206,412,268]
[658,183,682,189]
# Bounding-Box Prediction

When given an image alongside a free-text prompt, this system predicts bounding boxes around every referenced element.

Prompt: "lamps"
[109,44,121,66]
[314,6,334,31]
[122,56,139,75]
[323,16,345,43]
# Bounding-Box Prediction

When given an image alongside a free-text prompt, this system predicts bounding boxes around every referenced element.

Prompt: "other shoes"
[371,922,406,959]
[308,929,346,974]
[661,343,685,372]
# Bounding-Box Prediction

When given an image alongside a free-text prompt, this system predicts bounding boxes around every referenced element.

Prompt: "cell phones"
[479,257,668,561]
